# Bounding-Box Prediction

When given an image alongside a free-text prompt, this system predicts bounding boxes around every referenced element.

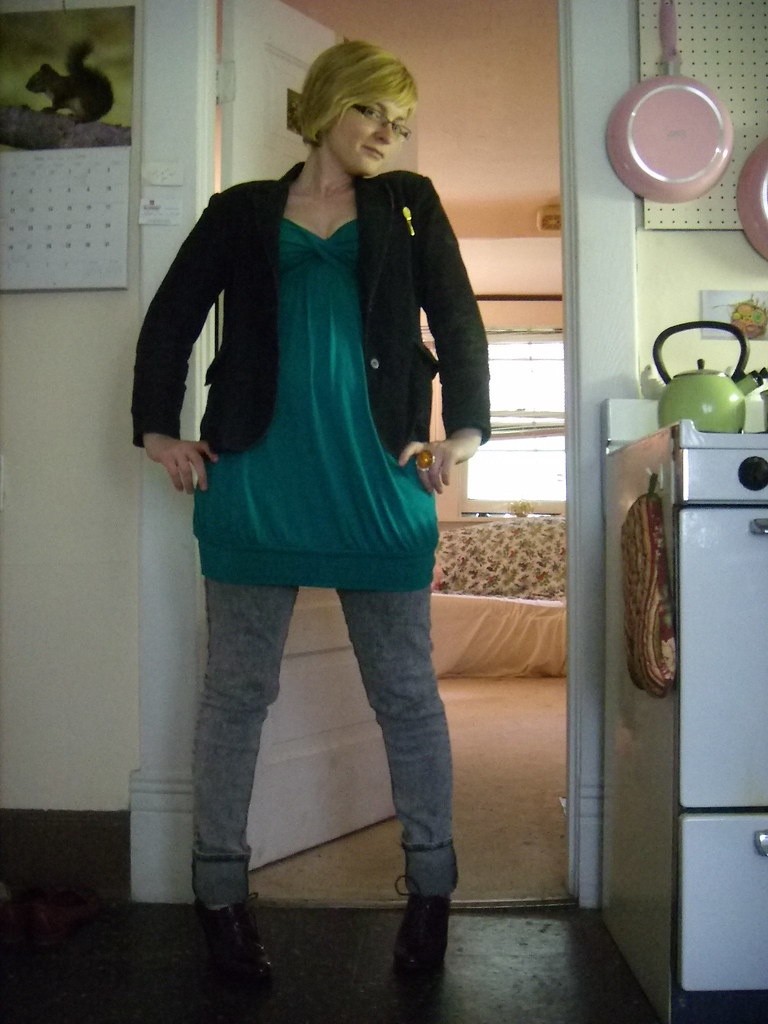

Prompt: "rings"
[415,450,433,471]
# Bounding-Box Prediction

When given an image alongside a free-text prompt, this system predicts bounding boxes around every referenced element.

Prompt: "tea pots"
[653,321,768,434]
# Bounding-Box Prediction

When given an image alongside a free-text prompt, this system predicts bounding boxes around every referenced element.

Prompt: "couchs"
[430,518,566,682]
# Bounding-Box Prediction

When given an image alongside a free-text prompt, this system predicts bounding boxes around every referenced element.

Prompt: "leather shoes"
[394,875,451,980]
[194,895,272,991]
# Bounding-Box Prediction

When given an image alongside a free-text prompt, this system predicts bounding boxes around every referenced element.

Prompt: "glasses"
[351,103,412,142]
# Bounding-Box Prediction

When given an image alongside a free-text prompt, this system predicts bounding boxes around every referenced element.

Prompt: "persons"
[132,43,487,983]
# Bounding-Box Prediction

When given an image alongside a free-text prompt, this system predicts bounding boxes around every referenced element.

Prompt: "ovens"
[600,422,768,1001]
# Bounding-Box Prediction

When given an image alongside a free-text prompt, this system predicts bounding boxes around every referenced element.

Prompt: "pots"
[738,138,768,261]
[605,0,734,204]
